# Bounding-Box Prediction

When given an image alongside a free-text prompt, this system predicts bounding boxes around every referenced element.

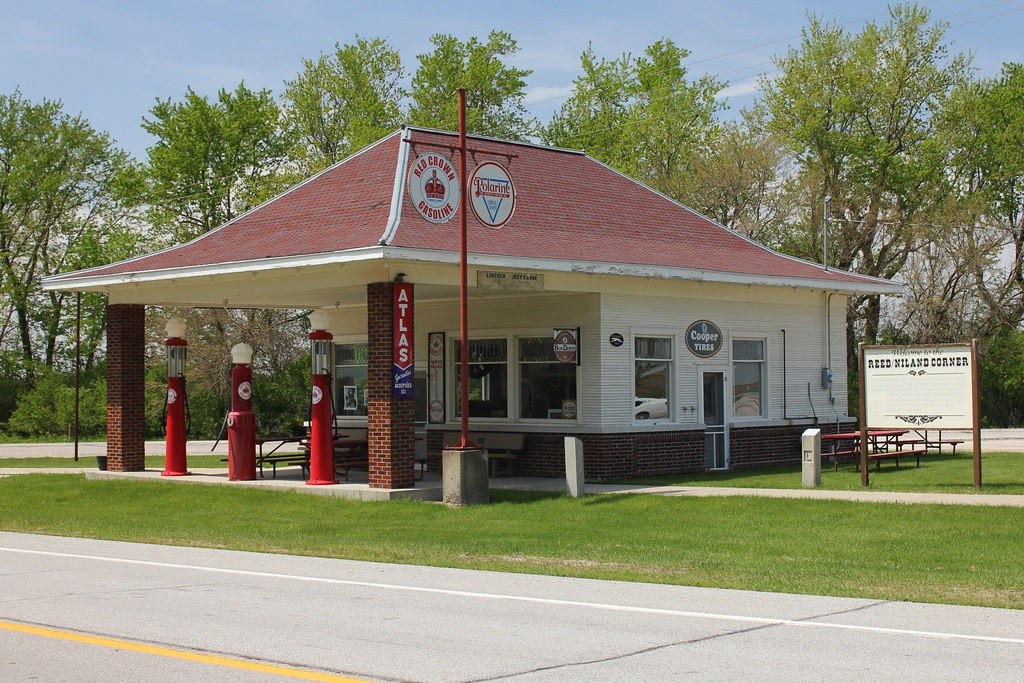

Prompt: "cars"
[634,396,667,420]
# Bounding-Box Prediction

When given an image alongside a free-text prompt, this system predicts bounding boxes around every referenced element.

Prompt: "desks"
[821,434,862,472]
[297,437,424,482]
[854,430,909,454]
[256,435,350,478]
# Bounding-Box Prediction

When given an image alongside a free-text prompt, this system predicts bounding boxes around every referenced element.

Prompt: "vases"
[96,456,107,471]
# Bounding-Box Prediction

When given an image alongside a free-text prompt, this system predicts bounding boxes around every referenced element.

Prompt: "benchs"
[821,440,964,472]
[426,430,524,478]
[220,453,429,482]
